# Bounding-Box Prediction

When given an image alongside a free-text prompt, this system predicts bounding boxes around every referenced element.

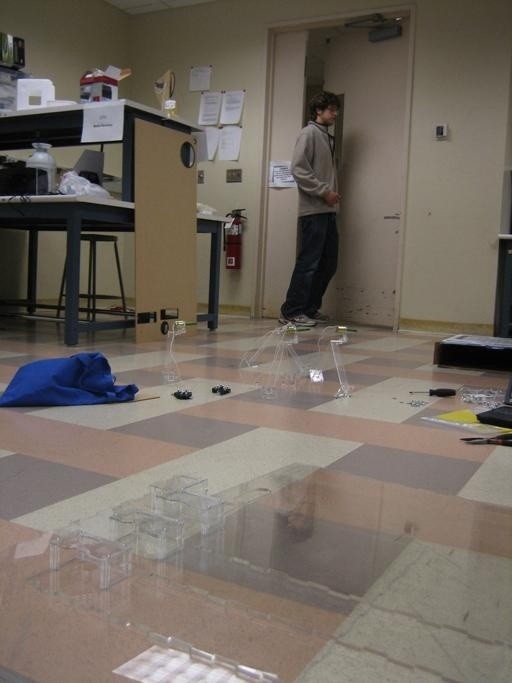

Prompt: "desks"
[1,192,133,346]
[196,213,232,328]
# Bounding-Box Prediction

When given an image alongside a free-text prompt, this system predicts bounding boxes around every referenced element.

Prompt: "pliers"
[460,434,512,446]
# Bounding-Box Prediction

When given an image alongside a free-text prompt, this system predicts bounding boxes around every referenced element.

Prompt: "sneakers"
[278,312,329,327]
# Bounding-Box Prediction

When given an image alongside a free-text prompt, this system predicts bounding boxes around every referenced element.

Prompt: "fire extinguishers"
[223,208,247,269]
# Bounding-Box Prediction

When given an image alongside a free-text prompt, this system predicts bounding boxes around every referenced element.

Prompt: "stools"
[59,233,125,320]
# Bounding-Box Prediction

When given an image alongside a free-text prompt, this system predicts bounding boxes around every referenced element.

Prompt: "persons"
[279,92,343,330]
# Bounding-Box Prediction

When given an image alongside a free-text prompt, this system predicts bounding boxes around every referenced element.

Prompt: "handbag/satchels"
[0,352,139,407]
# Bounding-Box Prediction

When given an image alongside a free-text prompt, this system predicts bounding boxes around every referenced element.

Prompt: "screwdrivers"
[409,388,455,396]
